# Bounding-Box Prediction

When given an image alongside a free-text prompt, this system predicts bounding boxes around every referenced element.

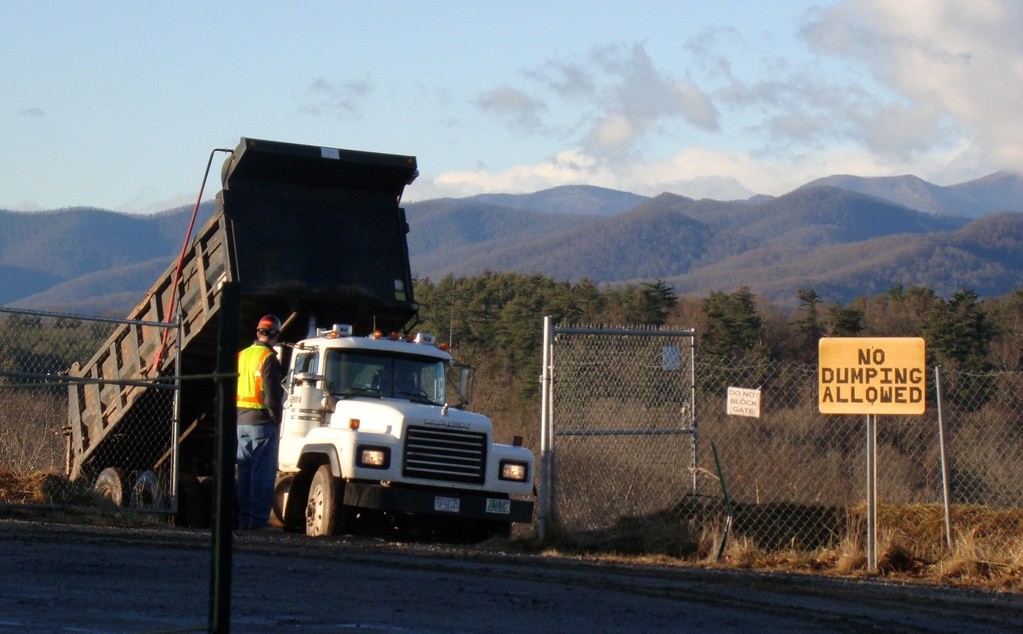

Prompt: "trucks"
[57,137,537,545]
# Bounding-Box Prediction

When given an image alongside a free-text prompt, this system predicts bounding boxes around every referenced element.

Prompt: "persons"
[236,315,284,535]
[383,367,396,384]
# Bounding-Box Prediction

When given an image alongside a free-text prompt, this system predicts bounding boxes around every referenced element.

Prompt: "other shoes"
[238,523,249,532]
[249,522,283,534]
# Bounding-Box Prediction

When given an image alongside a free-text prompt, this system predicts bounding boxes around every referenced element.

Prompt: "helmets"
[257,315,281,332]
